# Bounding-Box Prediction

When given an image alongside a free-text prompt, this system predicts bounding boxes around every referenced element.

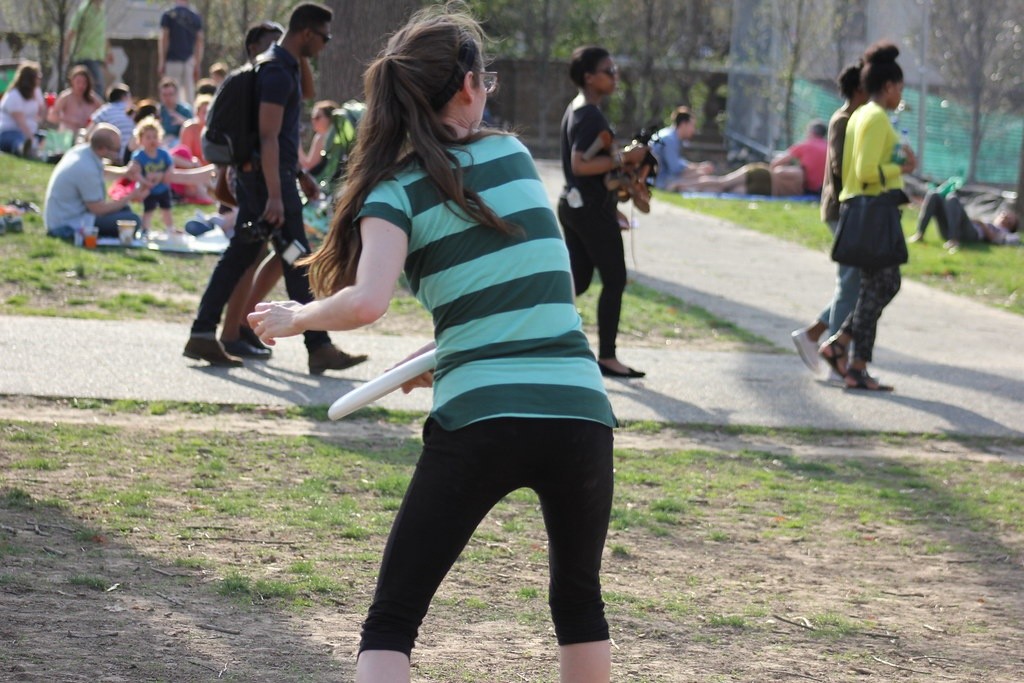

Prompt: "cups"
[117,221,137,245]
[84,227,99,249]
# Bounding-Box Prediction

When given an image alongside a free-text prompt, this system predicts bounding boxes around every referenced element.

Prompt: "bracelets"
[615,152,624,166]
[295,170,307,179]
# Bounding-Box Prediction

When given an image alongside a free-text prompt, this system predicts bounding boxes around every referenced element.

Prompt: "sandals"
[844,368,891,392]
[819,336,850,378]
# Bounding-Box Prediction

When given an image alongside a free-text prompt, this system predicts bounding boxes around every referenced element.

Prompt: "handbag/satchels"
[831,164,908,269]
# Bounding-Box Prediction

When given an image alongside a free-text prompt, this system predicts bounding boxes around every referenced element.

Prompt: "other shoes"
[791,329,819,375]
[596,359,646,378]
[308,342,368,375]
[815,363,849,386]
[220,335,272,358]
[184,334,242,367]
[239,324,266,348]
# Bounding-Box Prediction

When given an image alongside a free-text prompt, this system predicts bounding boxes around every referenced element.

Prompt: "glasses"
[307,26,332,44]
[581,64,616,78]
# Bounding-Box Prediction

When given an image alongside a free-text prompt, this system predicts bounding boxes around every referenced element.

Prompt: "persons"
[906,188,1022,246]
[128,120,178,237]
[246,0,620,683]
[62,0,114,97]
[791,38,915,391]
[306,100,342,180]
[195,77,219,95]
[179,94,215,166]
[645,105,713,189]
[85,82,135,164]
[0,60,45,156]
[668,161,805,194]
[47,65,106,137]
[43,122,142,240]
[182,2,369,375]
[769,119,827,194]
[555,44,652,376]
[158,76,193,137]
[209,63,228,82]
[123,100,217,204]
[156,0,205,105]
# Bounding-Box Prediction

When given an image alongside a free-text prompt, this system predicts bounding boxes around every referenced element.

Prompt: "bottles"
[890,118,898,132]
[897,128,910,159]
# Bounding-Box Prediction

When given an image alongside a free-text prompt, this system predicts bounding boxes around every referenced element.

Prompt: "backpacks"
[201,54,298,162]
